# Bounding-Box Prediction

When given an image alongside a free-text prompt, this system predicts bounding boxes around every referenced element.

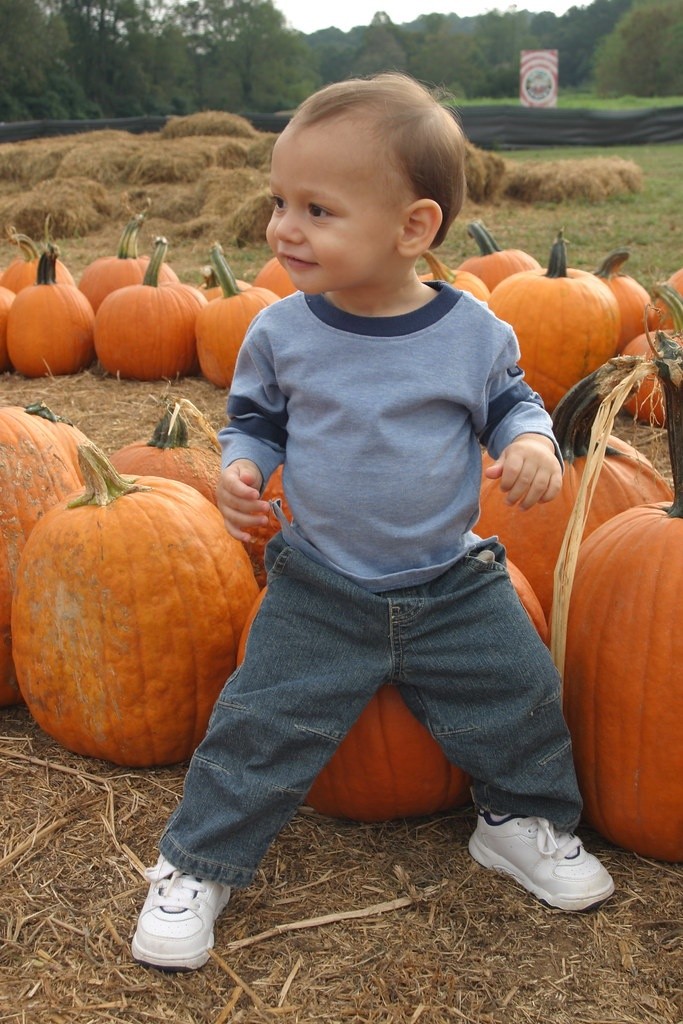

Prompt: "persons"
[131,74,615,967]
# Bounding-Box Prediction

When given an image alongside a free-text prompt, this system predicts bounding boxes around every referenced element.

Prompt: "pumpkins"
[0,398,290,768]
[235,219,683,863]
[0,214,301,389]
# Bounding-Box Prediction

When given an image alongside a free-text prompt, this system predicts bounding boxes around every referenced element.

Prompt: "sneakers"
[468,812,615,914]
[131,854,231,971]
